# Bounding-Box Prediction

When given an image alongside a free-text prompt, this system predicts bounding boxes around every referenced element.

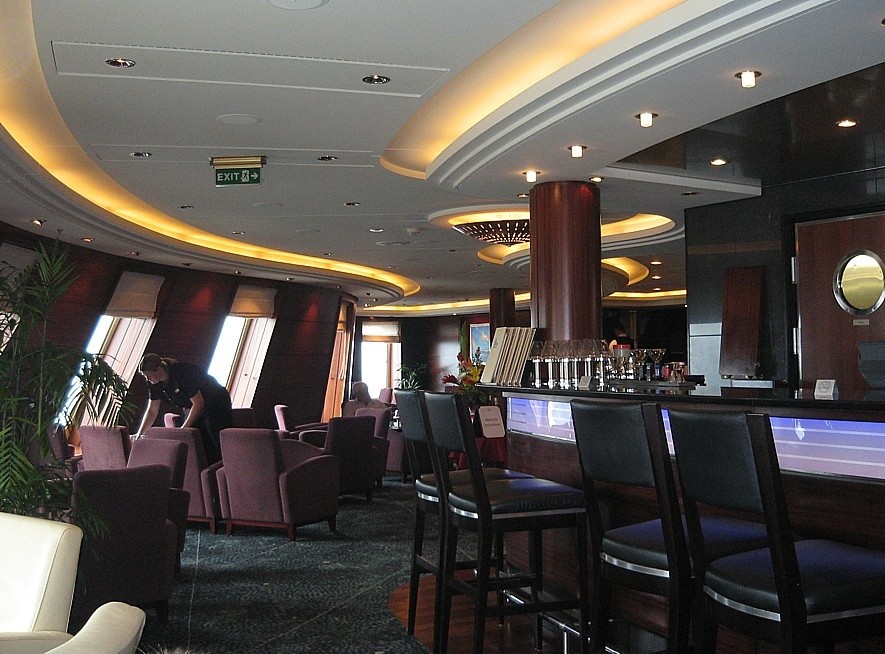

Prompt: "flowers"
[441,346,501,411]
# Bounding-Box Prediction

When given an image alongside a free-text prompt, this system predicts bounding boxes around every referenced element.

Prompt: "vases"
[472,402,495,438]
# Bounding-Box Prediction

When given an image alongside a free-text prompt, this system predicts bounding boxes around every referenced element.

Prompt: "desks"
[450,437,508,472]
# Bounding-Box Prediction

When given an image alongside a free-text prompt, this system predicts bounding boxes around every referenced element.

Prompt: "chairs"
[23,385,450,628]
[0,511,146,654]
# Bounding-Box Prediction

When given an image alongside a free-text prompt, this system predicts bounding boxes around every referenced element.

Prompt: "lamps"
[453,220,530,248]
[523,171,540,183]
[568,145,587,158]
[735,71,761,88]
[636,112,658,128]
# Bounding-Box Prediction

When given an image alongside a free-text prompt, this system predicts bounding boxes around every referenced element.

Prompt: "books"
[480,326,536,386]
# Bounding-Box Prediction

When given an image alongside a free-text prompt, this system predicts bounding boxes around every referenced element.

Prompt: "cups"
[529,339,609,358]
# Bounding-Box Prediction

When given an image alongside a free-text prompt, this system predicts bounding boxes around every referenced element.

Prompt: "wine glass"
[630,348,666,382]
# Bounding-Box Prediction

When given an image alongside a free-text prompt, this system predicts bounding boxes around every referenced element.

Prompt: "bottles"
[613,344,630,368]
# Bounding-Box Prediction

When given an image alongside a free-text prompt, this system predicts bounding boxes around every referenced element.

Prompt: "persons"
[342,381,386,417]
[135,353,232,463]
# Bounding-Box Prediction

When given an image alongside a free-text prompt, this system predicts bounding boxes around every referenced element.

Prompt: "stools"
[393,389,590,654]
[568,399,885,654]
